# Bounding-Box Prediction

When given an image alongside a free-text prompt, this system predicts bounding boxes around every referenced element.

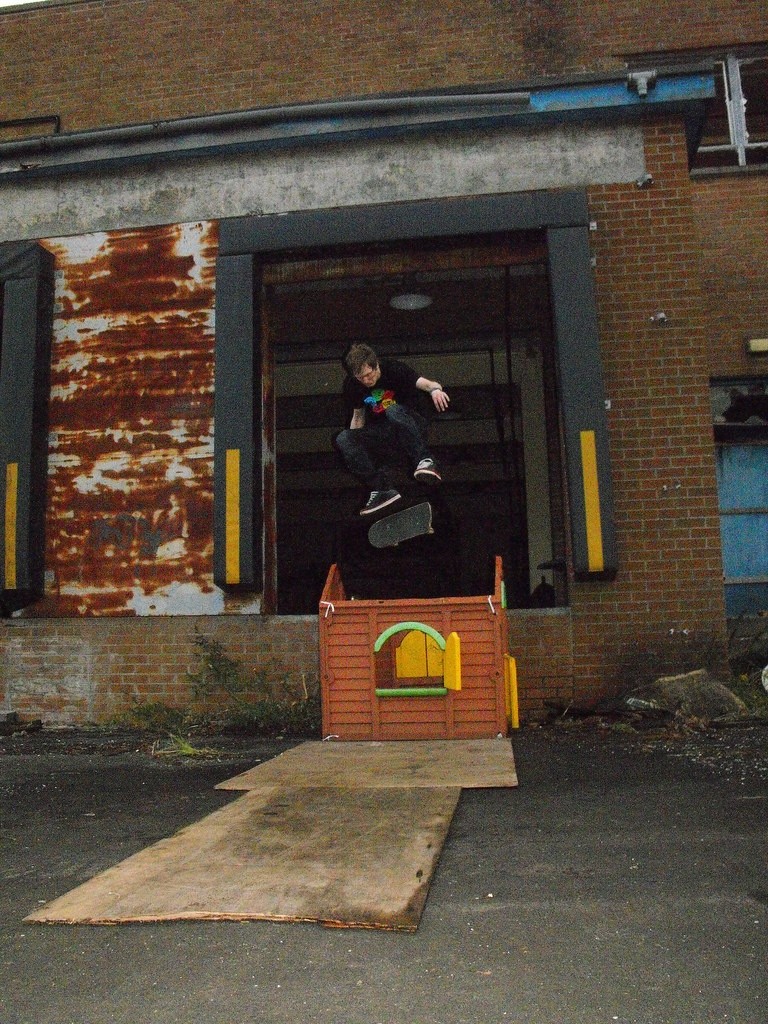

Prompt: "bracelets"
[428,387,441,395]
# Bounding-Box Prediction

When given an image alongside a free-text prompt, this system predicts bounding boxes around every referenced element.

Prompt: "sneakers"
[360,489,402,516]
[414,457,443,483]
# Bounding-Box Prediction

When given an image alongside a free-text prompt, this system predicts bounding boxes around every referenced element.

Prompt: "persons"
[334,344,451,516]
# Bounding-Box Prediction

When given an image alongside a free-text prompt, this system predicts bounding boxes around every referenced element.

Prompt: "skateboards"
[369,502,434,549]
